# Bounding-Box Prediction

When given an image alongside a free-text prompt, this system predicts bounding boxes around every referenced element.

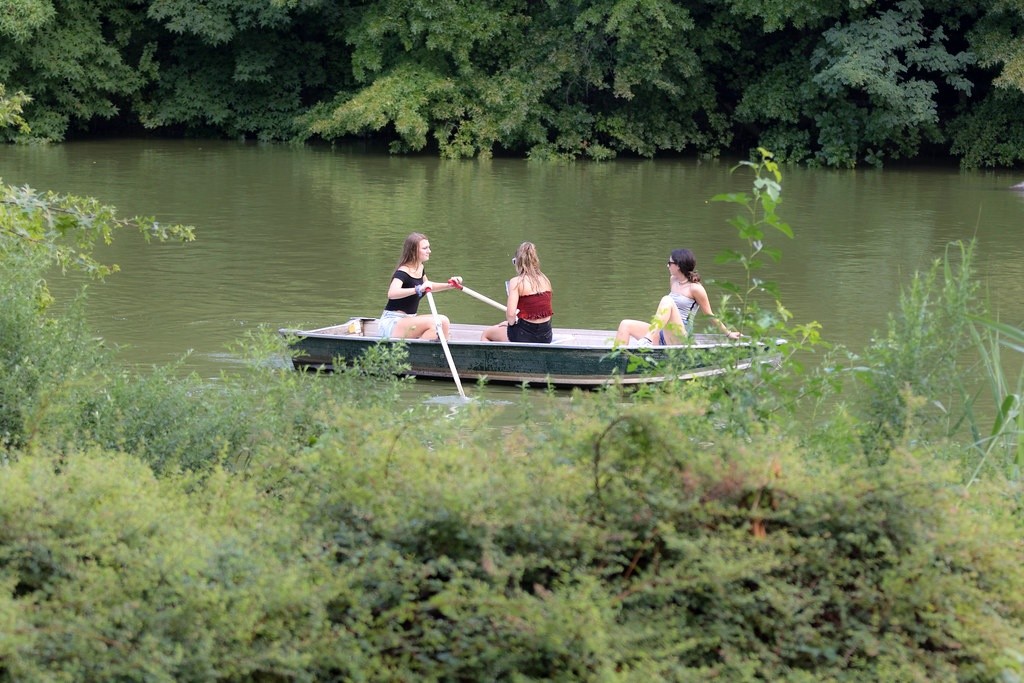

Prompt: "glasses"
[512,257,516,264]
[668,261,677,265]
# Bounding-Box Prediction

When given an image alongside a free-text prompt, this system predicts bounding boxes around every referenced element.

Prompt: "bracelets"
[727,332,731,337]
[415,285,422,297]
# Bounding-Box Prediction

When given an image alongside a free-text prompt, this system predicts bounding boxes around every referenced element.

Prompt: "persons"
[482,242,552,343]
[378,232,463,340]
[613,248,745,346]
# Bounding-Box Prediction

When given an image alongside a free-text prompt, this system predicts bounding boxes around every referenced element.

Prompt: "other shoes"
[635,337,653,346]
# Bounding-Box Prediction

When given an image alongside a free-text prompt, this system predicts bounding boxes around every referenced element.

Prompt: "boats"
[278,313,791,389]
